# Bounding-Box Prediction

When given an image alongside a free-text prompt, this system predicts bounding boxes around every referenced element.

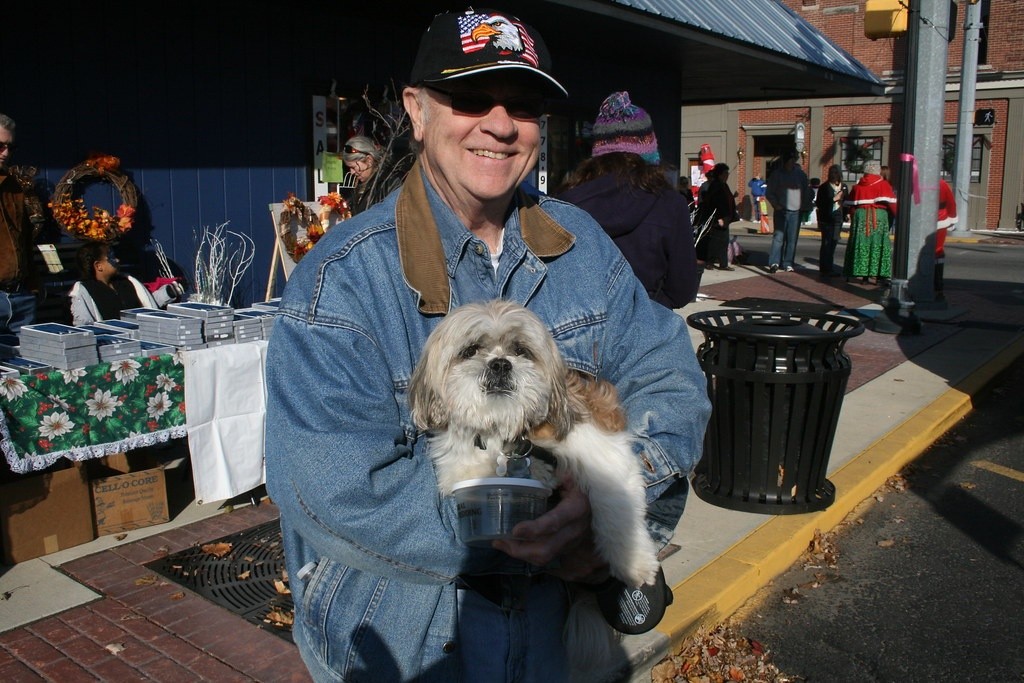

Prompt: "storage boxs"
[0,452,185,566]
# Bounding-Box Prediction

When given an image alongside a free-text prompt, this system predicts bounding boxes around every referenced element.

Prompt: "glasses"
[343,145,371,155]
[0,142,15,152]
[421,83,550,121]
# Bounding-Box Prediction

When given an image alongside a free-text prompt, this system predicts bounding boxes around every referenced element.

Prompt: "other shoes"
[718,266,735,271]
[706,264,715,270]
[786,266,795,272]
[770,264,779,273]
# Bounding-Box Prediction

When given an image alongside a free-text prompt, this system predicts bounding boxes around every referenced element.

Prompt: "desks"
[0,340,270,507]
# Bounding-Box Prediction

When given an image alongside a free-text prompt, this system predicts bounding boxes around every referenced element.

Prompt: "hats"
[591,90,661,164]
[411,9,569,97]
[863,161,881,175]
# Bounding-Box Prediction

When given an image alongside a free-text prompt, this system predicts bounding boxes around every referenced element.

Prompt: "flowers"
[320,194,353,219]
[84,151,121,175]
[280,194,323,257]
[48,191,137,239]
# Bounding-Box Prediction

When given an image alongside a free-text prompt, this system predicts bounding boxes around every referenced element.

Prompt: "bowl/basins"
[450,477,554,547]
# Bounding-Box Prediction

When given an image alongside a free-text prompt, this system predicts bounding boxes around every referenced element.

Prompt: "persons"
[558,91,704,316]
[342,136,402,217]
[68,240,177,327]
[0,114,62,337]
[262,0,713,683]
[678,146,957,290]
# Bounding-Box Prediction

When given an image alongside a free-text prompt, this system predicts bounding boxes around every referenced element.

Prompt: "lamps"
[802,147,807,165]
[737,147,743,165]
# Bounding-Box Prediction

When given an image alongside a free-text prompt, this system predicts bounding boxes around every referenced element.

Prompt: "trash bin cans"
[686,308,866,515]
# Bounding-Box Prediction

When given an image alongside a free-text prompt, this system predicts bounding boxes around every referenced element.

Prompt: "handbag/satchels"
[759,201,767,215]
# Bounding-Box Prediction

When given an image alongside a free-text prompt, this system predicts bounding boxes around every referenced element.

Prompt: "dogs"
[408,299,660,590]
